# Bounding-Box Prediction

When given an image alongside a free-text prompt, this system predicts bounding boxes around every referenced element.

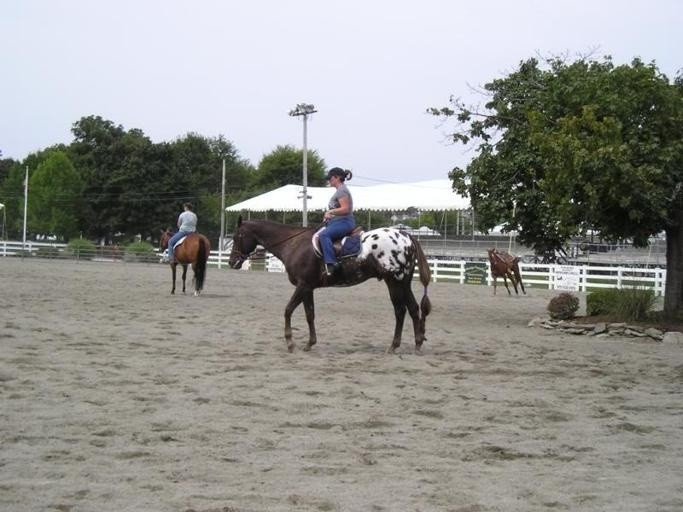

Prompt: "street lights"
[288,102,317,228]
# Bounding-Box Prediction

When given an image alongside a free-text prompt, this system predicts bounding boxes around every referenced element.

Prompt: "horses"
[226,213,431,355]
[157,226,211,297]
[486,247,527,296]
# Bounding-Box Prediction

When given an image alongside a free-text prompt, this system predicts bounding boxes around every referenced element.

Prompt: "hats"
[325,167,345,180]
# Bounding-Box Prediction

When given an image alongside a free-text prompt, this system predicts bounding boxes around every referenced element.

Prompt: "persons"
[166,202,198,263]
[319,167,355,277]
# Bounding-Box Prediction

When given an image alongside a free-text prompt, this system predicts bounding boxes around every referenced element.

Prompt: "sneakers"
[321,268,334,277]
